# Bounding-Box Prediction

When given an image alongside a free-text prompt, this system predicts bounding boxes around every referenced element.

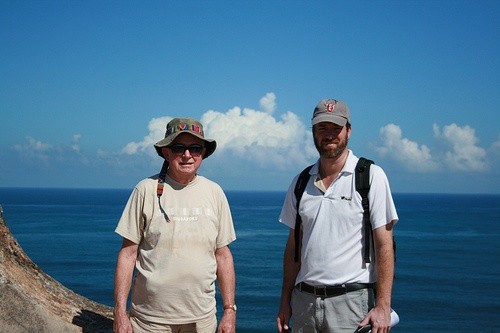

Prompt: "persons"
[113,117,238,333]
[277,97,400,333]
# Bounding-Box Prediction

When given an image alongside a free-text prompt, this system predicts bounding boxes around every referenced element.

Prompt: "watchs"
[223,304,237,311]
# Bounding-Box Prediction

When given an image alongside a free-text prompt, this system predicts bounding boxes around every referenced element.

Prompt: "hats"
[154,118,217,160]
[311,98,350,127]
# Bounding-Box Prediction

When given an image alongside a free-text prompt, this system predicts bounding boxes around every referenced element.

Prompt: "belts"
[297,281,374,296]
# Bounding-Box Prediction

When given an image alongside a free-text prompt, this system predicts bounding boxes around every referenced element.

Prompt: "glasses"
[163,142,206,154]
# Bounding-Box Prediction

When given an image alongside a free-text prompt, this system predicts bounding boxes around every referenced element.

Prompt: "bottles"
[354,307,400,333]
